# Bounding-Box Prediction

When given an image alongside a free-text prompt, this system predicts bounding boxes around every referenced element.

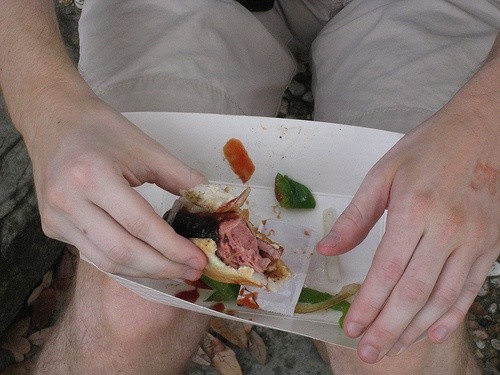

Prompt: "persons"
[0,0,500,375]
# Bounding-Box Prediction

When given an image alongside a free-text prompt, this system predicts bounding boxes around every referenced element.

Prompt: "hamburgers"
[162,184,294,294]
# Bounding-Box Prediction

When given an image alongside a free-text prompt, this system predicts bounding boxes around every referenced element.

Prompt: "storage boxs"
[77,111,428,351]
